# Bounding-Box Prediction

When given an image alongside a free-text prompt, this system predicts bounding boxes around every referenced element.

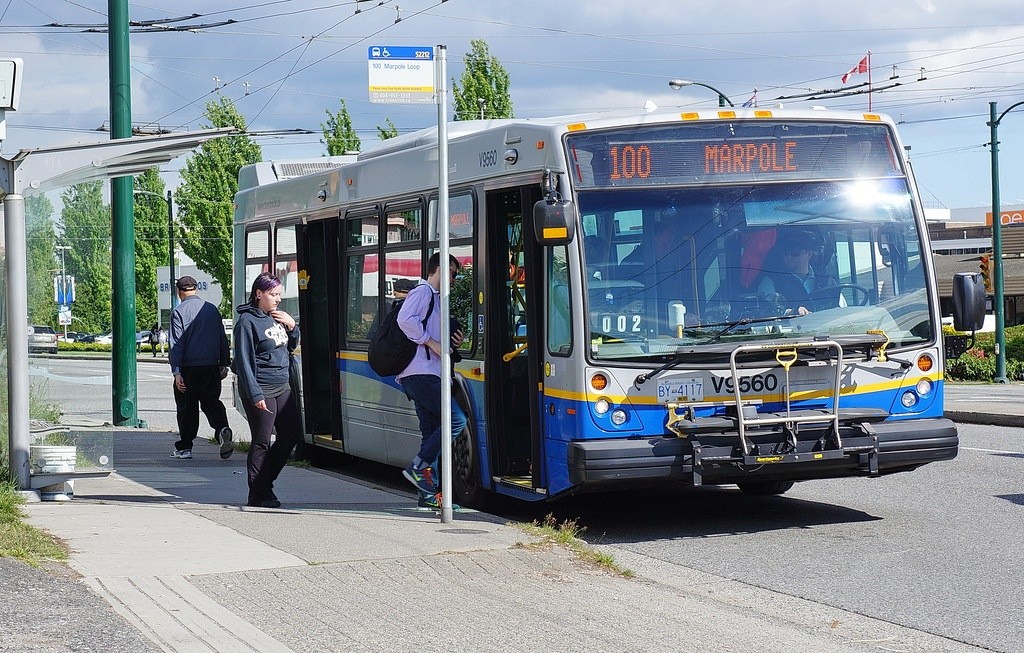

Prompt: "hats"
[394,279,415,294]
[176,276,197,291]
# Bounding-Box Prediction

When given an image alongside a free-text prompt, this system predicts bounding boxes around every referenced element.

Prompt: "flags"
[840,56,869,84]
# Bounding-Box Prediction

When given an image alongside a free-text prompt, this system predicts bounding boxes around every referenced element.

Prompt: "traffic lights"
[980,254,992,291]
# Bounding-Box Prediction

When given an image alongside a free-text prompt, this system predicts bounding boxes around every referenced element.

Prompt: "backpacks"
[150,332,158,342]
[368,284,434,376]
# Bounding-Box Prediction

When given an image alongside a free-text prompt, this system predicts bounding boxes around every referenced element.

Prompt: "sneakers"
[169,449,192,458]
[403,462,436,494]
[219,426,233,459]
[417,493,460,510]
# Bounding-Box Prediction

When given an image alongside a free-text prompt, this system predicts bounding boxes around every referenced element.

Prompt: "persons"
[757,230,848,316]
[148,324,167,357]
[169,276,234,460]
[367,278,417,340]
[395,252,467,512]
[233,272,302,508]
[135,327,142,356]
[509,221,675,350]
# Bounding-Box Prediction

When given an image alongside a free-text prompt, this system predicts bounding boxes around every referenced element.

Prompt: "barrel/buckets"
[31,445,76,502]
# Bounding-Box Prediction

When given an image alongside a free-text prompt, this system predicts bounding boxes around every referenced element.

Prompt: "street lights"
[55,246,73,341]
[174,249,184,279]
[144,190,176,311]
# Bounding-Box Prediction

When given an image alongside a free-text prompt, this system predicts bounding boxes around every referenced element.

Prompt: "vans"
[941,315,996,335]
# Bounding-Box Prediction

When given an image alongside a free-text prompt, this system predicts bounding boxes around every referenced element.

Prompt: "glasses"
[450,269,457,278]
[782,248,807,257]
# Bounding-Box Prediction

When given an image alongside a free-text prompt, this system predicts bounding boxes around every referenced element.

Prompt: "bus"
[230,106,986,505]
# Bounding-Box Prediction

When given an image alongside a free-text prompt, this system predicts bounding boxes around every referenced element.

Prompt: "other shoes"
[248,488,281,508]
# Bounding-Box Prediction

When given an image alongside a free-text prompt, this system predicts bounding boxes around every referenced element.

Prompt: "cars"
[57,331,86,343]
[73,336,99,343]
[141,331,151,342]
[28,325,58,354]
[95,330,112,344]
